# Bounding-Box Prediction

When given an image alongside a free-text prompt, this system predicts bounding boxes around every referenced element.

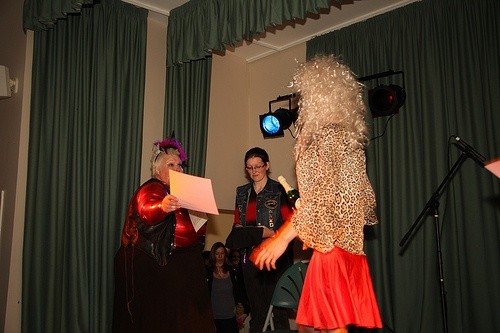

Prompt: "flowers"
[153,137,188,161]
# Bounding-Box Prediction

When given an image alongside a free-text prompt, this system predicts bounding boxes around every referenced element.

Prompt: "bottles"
[277,176,302,211]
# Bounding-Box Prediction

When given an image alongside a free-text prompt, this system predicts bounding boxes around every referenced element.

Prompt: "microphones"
[455,136,488,168]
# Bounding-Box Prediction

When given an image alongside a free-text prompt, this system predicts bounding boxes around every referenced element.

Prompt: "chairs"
[259,261,309,333]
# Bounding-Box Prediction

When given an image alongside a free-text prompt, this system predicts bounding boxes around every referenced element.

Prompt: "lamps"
[259,99,292,139]
[368,72,405,118]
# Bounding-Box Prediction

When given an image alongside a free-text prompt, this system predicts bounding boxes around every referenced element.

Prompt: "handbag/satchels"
[131,239,163,278]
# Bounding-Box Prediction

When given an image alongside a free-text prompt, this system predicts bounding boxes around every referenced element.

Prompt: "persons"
[254,53,383,333]
[204,242,244,333]
[112,137,209,333]
[223,147,294,333]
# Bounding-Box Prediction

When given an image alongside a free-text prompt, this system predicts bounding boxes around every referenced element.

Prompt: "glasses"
[246,163,265,170]
